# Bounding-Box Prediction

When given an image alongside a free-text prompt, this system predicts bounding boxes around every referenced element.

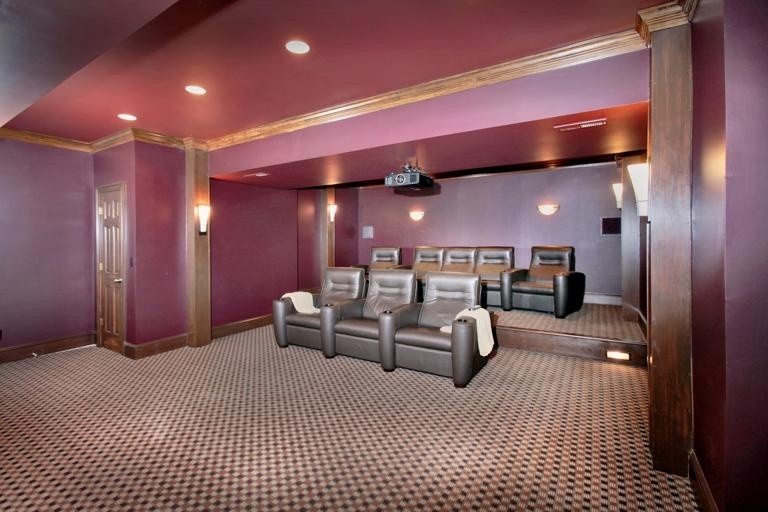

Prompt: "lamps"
[197,204,210,235]
[611,162,649,217]
[329,204,337,224]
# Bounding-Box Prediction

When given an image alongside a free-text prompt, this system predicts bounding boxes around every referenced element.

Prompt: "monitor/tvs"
[600,216,621,237]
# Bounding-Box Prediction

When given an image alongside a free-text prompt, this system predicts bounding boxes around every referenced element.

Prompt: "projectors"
[384,161,434,191]
